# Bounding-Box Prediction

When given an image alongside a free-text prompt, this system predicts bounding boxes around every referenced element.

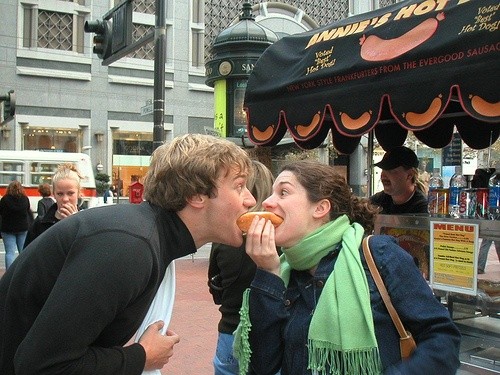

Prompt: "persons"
[0,133,258,374]
[22,162,89,249]
[0,181,34,270]
[207,161,276,374]
[478,239,500,275]
[246,161,462,375]
[368,146,429,215]
[37,183,54,217]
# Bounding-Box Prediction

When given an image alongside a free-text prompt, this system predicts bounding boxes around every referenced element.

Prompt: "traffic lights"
[3,90,15,119]
[84,19,109,58]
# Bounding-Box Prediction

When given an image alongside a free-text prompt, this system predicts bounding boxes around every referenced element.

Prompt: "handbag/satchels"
[363,234,418,361]
[209,273,225,305]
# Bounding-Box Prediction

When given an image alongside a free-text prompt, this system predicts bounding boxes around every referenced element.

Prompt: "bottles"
[487,162,500,221]
[448,166,468,219]
[426,168,445,217]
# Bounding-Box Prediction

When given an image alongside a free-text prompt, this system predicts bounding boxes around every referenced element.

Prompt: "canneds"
[429,188,439,216]
[458,187,475,220]
[440,188,450,216]
[475,187,492,218]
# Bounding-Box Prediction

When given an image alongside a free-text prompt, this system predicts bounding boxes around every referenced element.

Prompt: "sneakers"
[477,268,485,274]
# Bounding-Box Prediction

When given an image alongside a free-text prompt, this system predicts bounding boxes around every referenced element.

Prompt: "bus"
[0,150,96,218]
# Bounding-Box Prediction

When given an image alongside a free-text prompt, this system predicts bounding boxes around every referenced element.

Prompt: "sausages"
[400,93,442,128]
[291,113,321,138]
[339,110,372,131]
[356,10,447,62]
[465,93,498,119]
[249,123,274,141]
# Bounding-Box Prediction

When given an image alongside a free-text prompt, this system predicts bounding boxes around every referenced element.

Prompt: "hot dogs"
[236,209,284,235]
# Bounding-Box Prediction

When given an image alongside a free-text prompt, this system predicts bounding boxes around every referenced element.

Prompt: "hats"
[369,146,419,170]
